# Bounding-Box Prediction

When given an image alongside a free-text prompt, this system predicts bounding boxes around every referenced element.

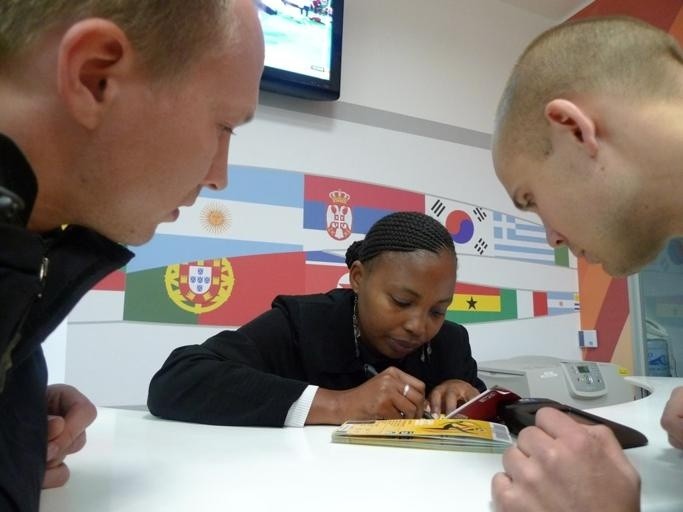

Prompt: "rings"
[403,385,409,397]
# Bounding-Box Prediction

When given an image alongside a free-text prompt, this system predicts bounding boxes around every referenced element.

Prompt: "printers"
[469,354,634,407]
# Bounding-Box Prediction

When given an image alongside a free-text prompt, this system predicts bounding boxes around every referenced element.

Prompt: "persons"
[488,14,683,512]
[0,0,266,512]
[147,211,487,430]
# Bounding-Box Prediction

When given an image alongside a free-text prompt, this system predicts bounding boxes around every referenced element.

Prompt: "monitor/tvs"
[254,0,346,102]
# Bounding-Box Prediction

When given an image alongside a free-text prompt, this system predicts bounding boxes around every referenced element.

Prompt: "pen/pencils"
[362,361,434,419]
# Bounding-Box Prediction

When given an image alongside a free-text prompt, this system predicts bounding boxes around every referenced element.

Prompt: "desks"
[40,374,683,511]
